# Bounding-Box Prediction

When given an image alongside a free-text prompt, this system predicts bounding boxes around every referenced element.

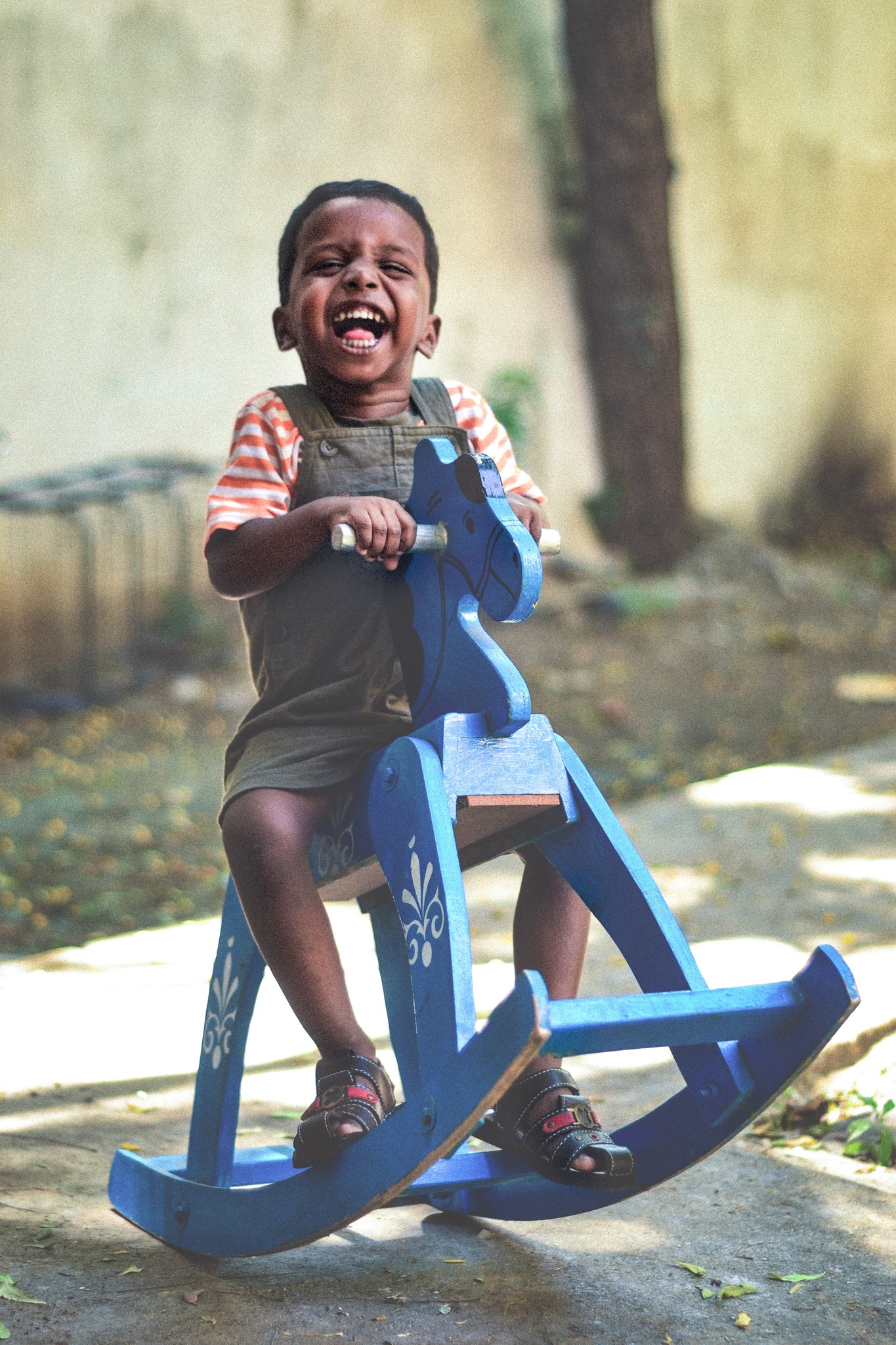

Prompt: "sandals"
[470,1067,636,1187]
[292,1050,395,1167]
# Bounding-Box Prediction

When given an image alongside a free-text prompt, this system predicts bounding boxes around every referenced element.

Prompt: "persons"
[203,178,641,1187]
